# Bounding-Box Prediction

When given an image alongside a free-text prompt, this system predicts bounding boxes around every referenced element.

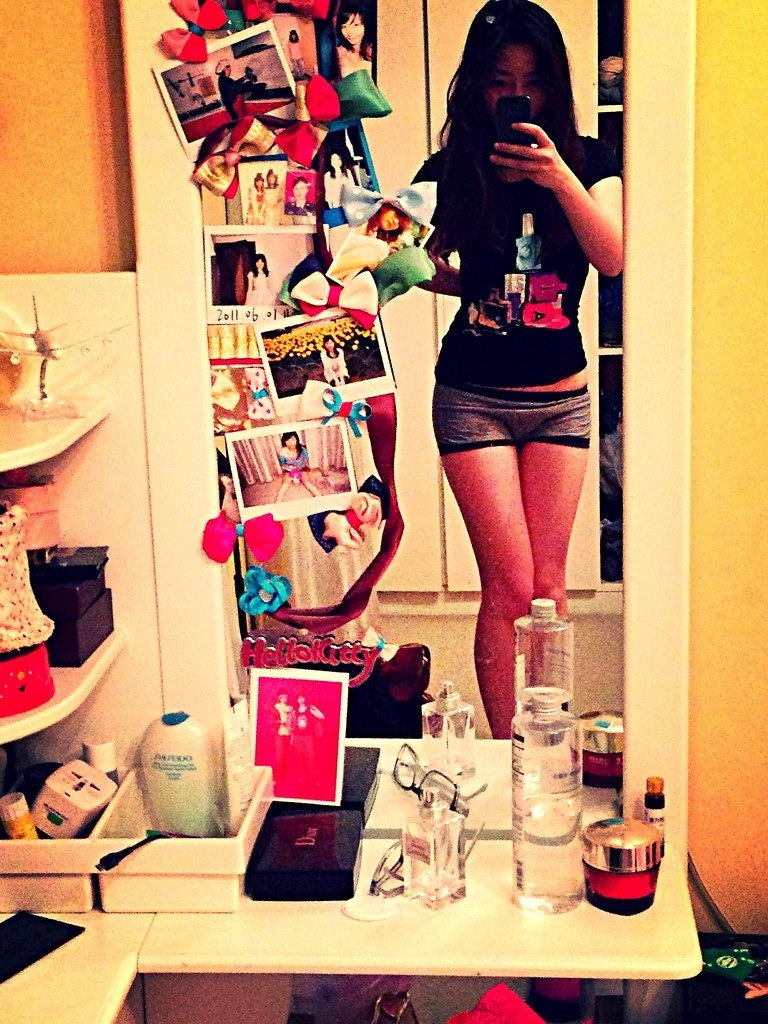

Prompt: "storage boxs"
[45,588,114,667]
[31,569,106,624]
[0,765,274,914]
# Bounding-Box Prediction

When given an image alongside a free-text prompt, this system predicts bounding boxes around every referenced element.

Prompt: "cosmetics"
[402,787,468,908]
[512,598,577,718]
[509,685,584,914]
[222,699,256,840]
[582,819,661,916]
[140,712,217,838]
[420,681,476,784]
[573,708,627,789]
[82,734,120,786]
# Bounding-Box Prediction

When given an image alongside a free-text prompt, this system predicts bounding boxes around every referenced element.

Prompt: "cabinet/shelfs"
[598,0,626,590]
[0,393,122,745]
[361,0,600,594]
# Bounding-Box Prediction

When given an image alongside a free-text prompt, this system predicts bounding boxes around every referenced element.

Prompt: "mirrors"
[199,0,627,842]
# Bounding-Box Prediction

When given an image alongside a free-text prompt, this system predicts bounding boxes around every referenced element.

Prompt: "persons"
[272,431,323,503]
[407,0,623,738]
[319,334,351,388]
[243,253,278,306]
[218,3,374,225]
[267,689,325,783]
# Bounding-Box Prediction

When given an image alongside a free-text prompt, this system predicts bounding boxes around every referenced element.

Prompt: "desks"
[137,842,703,1024]
